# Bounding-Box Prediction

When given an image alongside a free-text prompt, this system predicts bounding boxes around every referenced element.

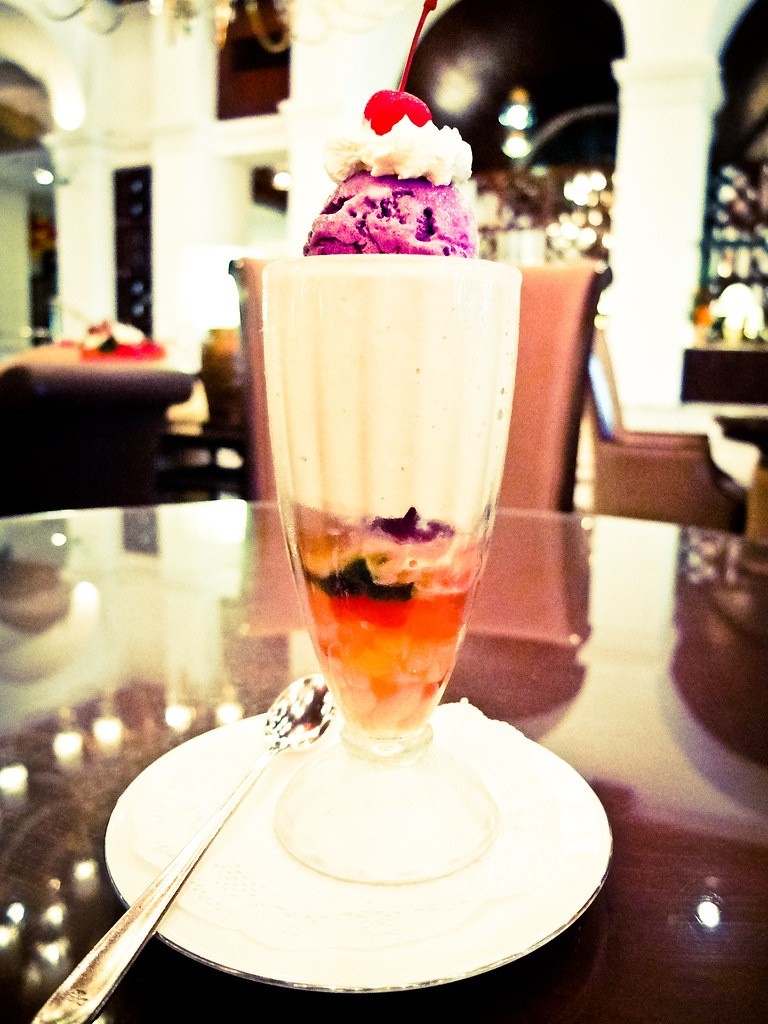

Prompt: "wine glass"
[258,256,522,884]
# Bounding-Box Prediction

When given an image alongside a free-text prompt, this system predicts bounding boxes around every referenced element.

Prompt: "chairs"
[586,370,746,530]
[231,264,603,511]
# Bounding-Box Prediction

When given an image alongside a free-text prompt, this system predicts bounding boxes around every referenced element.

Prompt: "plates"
[105,702,612,994]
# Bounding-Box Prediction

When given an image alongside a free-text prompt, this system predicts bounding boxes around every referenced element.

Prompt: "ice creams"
[261,0,520,761]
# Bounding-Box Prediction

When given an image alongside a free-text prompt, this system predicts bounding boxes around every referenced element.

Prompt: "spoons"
[31,675,332,1024]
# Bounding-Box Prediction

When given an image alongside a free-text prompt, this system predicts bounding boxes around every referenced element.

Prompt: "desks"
[0,503,768,1024]
